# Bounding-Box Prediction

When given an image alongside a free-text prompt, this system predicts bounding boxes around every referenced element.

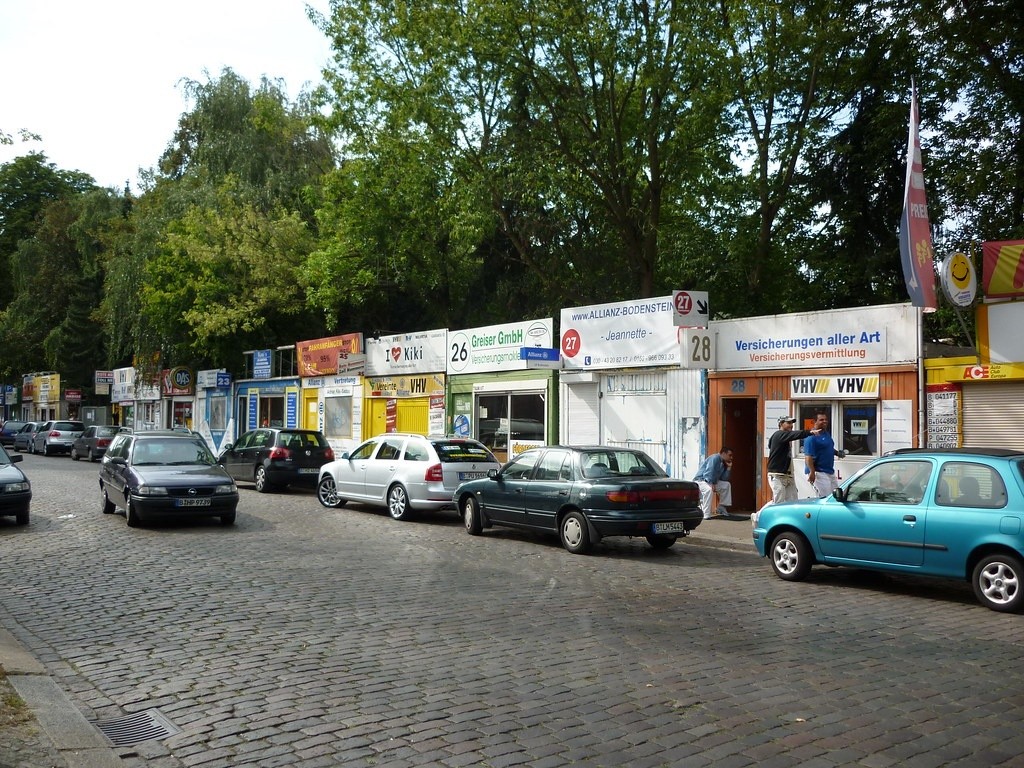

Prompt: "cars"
[751,447,1024,612]
[217,428,335,494]
[71,425,127,463]
[452,445,703,554]
[0,443,32,526]
[13,422,46,454]
[0,421,29,446]
[32,420,86,456]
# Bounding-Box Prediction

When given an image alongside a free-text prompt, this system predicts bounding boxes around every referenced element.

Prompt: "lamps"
[373,330,400,340]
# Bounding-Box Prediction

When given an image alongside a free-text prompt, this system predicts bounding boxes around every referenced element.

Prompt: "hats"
[777,416,796,427]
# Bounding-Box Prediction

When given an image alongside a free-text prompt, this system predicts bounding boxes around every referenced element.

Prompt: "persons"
[111,410,120,426]
[692,447,733,520]
[751,415,822,530]
[804,411,846,499]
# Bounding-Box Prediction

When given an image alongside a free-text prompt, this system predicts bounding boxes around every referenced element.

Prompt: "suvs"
[98,426,239,528]
[317,433,504,521]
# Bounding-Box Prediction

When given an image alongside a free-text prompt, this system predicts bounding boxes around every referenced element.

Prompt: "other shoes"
[705,513,711,519]
[717,505,731,516]
[751,513,758,530]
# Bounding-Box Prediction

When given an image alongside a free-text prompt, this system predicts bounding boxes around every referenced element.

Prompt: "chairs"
[591,463,607,473]
[953,477,979,503]
[288,440,301,447]
[939,479,950,502]
